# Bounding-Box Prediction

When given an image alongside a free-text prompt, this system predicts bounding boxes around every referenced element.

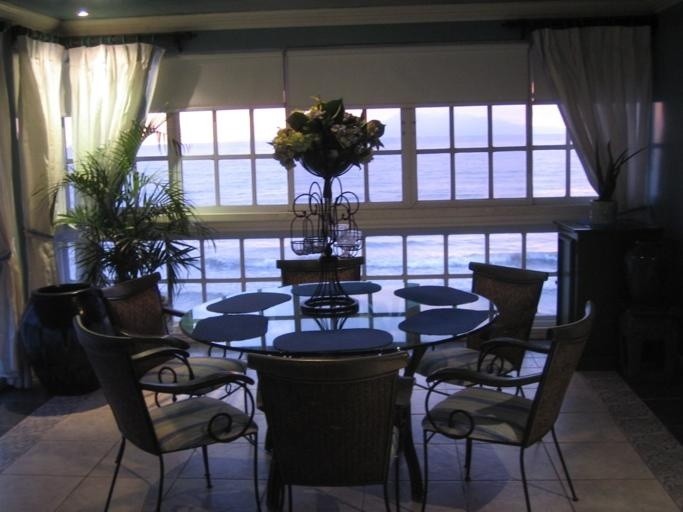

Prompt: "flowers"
[264,95,386,171]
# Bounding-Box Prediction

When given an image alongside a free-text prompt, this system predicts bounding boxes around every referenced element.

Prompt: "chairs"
[421,300,596,512]
[414,261,549,396]
[98,272,247,398]
[246,350,414,511]
[72,315,263,512]
[275,257,365,287]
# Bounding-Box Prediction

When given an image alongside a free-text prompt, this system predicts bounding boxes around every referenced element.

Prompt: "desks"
[178,278,502,511]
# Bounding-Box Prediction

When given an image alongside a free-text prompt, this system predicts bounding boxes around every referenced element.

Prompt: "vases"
[623,238,674,305]
[288,144,364,316]
[19,282,112,396]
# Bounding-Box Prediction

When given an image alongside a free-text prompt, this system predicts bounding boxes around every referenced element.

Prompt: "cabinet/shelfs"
[551,218,664,372]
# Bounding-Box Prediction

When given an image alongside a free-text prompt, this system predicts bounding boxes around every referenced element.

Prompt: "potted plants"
[590,139,648,226]
